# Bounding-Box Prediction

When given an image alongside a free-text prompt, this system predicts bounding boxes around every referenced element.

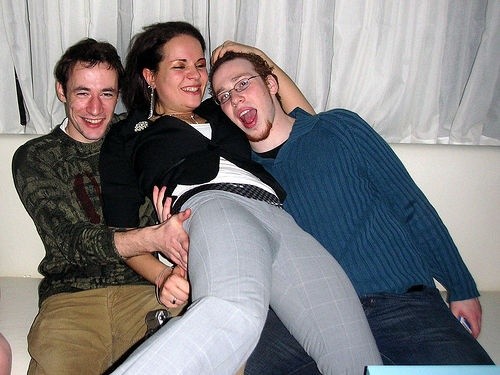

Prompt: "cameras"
[145,309,169,335]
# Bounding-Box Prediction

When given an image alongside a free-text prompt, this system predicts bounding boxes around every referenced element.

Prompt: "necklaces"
[169,112,200,124]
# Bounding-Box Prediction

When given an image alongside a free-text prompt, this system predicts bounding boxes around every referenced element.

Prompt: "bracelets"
[155,266,174,305]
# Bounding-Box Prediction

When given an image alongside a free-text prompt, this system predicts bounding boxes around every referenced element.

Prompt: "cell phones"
[460,315,472,335]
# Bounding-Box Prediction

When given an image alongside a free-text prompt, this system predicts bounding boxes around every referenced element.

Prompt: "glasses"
[213,74,263,106]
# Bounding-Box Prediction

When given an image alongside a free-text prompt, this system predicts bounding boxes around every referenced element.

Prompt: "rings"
[172,298,177,304]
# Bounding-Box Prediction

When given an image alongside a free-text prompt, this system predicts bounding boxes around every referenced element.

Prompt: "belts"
[170,182,284,214]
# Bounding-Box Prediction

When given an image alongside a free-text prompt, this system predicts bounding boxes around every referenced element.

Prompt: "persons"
[207,51,495,375]
[11,39,246,375]
[98,21,383,375]
